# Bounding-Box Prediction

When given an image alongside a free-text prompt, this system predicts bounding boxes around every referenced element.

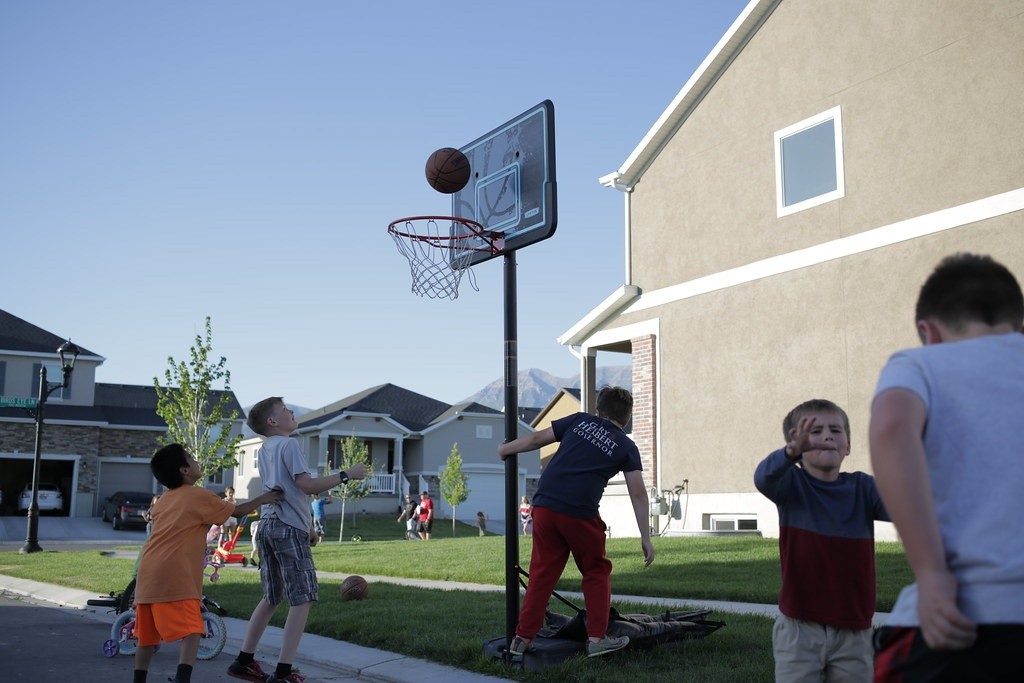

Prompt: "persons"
[754,400,892,683]
[226,397,368,683]
[498,387,654,657]
[218,488,237,547]
[472,512,486,536]
[312,490,333,543]
[873,252,1024,683]
[134,443,282,683]
[250,521,259,565]
[397,495,418,530]
[142,496,160,536]
[519,497,532,535]
[416,491,434,539]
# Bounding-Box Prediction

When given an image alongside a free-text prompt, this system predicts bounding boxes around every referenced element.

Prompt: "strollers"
[210,509,260,568]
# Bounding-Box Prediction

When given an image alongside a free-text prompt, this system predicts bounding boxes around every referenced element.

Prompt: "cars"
[102,491,155,531]
[18,481,64,517]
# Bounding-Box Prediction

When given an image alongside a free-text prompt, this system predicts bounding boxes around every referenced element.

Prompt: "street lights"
[18,337,83,556]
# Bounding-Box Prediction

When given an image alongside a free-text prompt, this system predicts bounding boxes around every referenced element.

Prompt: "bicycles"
[101,590,226,660]
[86,575,229,619]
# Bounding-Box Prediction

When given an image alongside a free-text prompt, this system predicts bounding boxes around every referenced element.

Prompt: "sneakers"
[586,634,629,657]
[227,660,270,682]
[266,672,304,683]
[510,636,533,655]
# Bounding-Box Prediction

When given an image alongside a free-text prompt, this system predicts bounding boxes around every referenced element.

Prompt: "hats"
[418,491,427,495]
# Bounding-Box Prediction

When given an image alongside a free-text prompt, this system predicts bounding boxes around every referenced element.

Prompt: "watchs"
[339,470,349,485]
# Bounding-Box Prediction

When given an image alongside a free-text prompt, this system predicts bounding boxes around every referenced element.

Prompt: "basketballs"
[425,147,471,194]
[341,575,368,601]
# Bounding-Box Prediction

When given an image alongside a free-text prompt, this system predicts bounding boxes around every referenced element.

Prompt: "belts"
[263,515,278,519]
